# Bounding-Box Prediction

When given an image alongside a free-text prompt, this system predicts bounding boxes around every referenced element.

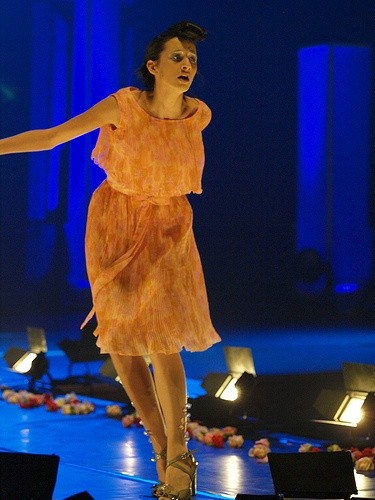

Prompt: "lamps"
[4,327,50,381]
[202,346,259,405]
[313,363,375,430]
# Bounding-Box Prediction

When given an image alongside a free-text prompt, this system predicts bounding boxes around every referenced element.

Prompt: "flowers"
[105,405,143,430]
[248,438,270,464]
[186,422,244,447]
[1,389,95,415]
[297,443,375,472]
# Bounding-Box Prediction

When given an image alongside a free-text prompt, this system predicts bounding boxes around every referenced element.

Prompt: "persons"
[0,22,222,499]
